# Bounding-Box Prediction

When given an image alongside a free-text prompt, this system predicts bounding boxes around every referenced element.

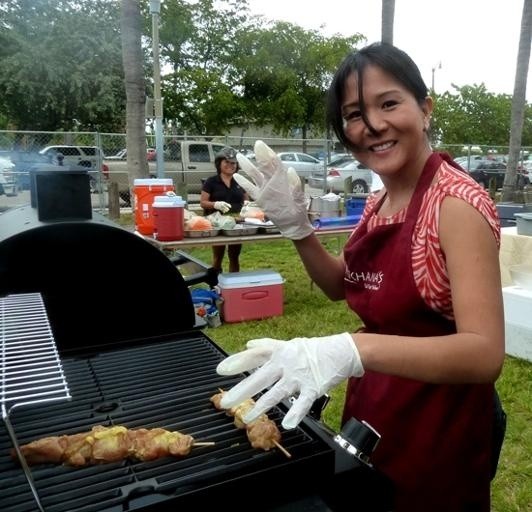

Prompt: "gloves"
[231,141,316,241]
[216,331,364,429]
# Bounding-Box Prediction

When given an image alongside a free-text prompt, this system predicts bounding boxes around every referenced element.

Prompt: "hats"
[215,146,239,163]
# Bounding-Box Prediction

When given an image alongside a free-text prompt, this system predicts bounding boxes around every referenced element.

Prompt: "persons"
[213,42,507,511]
[199,146,251,273]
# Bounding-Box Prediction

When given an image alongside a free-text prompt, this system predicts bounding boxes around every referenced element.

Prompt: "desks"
[133,225,357,291]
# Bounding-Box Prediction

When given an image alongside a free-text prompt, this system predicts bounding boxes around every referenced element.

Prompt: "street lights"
[432,61,443,91]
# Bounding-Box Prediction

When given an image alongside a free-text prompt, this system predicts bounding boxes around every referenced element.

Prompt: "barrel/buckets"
[152,195,187,241]
[132,178,173,235]
[306,195,341,224]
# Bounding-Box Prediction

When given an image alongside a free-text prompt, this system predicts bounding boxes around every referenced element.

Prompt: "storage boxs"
[498,212,532,363]
[217,268,286,323]
